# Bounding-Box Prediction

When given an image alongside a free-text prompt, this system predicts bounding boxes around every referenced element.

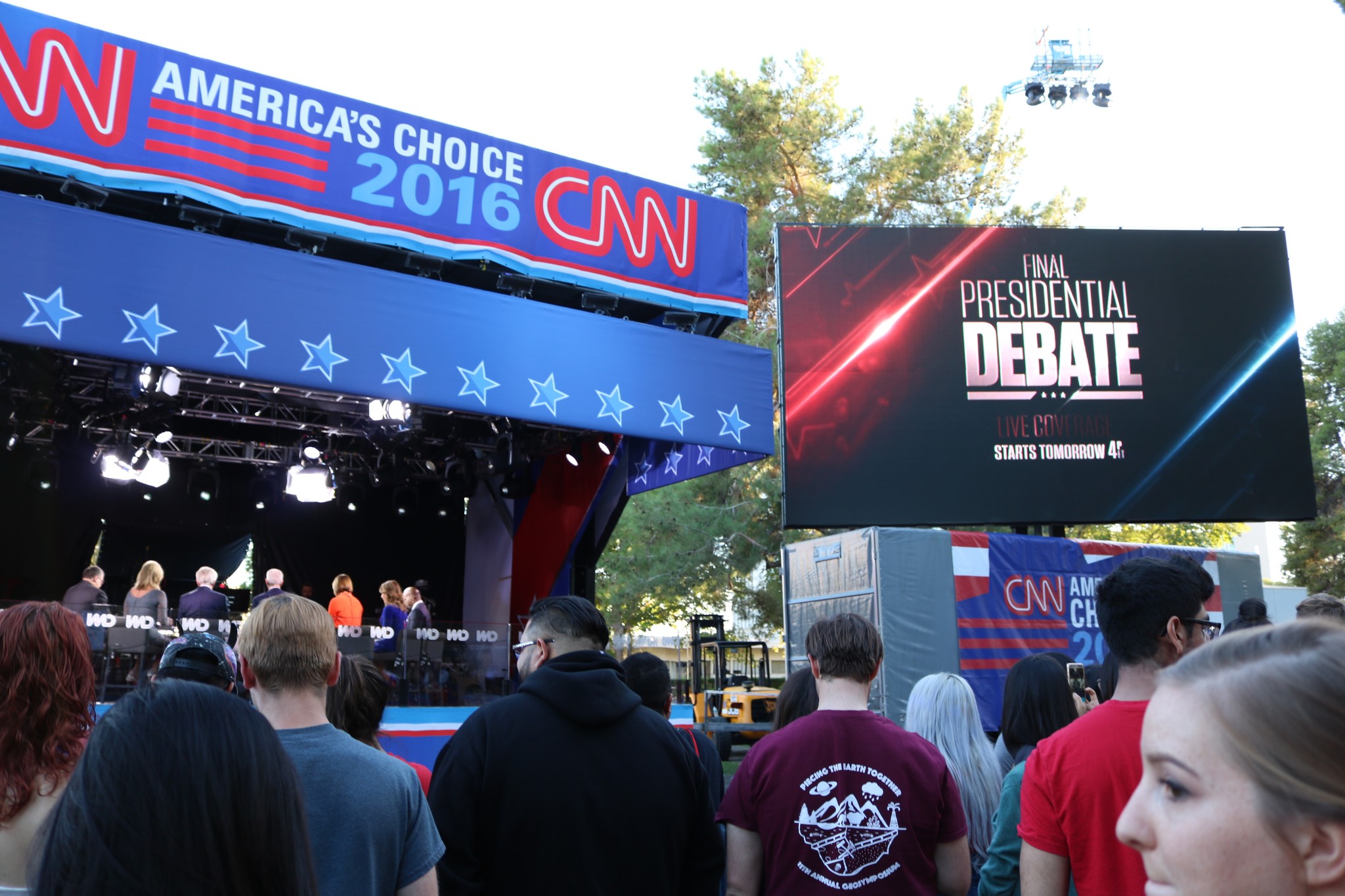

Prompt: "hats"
[157,631,239,696]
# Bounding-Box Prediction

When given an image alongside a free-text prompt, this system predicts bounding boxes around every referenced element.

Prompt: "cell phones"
[1067,662,1085,705]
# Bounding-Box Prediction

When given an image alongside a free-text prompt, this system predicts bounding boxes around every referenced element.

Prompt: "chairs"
[77,611,225,702]
[340,625,444,708]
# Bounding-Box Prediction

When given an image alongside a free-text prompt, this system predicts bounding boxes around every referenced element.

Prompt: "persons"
[0,556,1345,896]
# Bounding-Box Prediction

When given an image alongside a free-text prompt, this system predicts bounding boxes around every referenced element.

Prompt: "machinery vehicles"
[685,614,785,760]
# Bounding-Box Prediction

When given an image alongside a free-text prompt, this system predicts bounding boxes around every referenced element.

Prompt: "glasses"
[512,639,555,659]
[1160,618,1221,643]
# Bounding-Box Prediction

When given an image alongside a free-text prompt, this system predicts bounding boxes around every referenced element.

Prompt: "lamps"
[7,359,532,505]
[566,439,583,466]
[598,432,616,455]
